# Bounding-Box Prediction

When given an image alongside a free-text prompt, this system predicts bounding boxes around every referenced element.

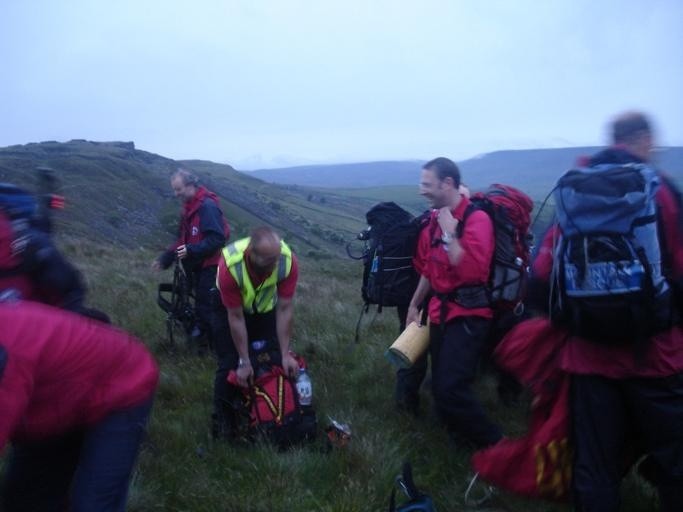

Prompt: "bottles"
[295,368,313,406]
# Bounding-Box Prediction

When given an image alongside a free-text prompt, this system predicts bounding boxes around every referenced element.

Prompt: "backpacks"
[347,201,428,343]
[457,182,533,328]
[549,164,678,342]
[222,350,332,457]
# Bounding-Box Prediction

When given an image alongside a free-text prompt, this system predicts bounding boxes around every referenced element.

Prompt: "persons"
[405,157,508,452]
[206,226,300,436]
[526,109,683,512]
[151,169,229,319]
[394,180,470,415]
[0,291,159,512]
[494,316,558,412]
